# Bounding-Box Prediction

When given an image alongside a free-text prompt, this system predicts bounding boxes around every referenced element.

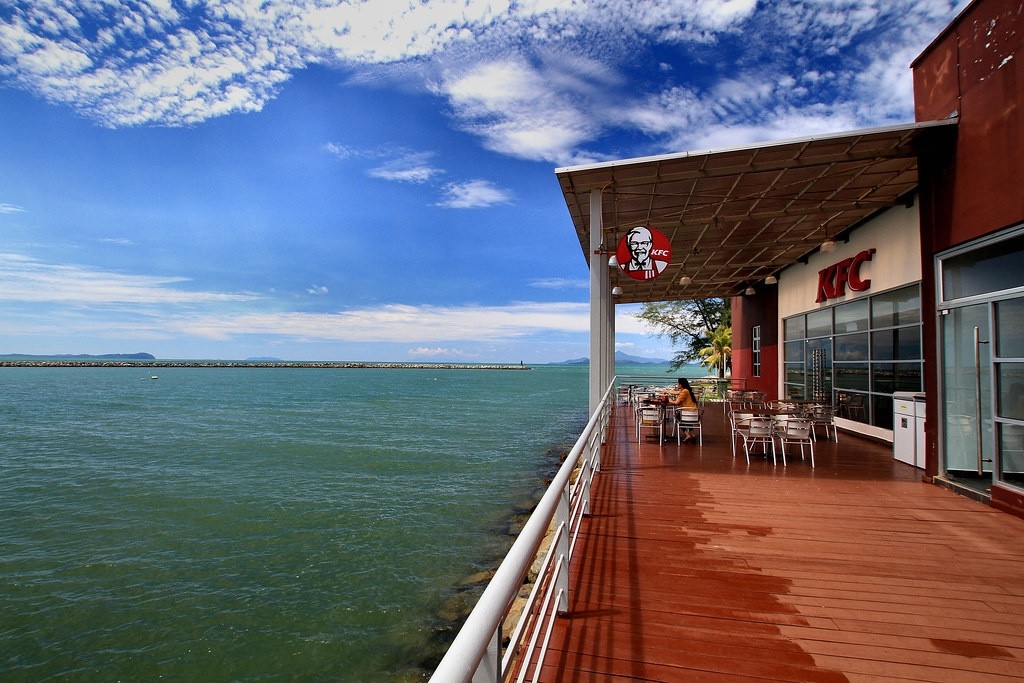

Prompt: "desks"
[734,410,801,460]
[776,399,827,403]
[619,382,643,406]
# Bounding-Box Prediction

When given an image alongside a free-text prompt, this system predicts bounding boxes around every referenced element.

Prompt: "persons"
[663,378,702,442]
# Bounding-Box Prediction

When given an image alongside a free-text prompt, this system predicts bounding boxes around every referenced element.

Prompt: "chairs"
[838,394,867,419]
[722,388,838,469]
[617,386,704,448]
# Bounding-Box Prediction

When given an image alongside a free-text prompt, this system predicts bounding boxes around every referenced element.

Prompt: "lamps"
[612,278,623,295]
[765,276,778,285]
[679,263,692,285]
[609,255,619,266]
[819,227,836,253]
[745,286,756,295]
